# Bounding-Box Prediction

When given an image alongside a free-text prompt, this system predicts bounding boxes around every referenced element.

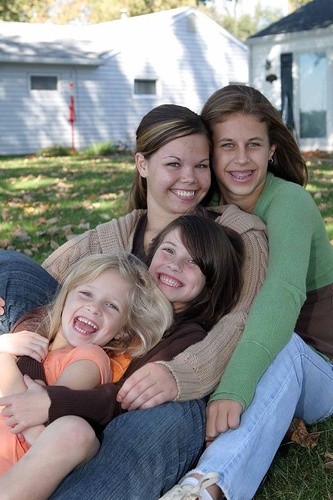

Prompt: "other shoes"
[158,476,214,500]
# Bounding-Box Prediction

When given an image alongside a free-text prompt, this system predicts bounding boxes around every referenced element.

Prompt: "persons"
[0,103,269,500]
[1,214,245,500]
[0,252,174,482]
[160,83,333,499]
